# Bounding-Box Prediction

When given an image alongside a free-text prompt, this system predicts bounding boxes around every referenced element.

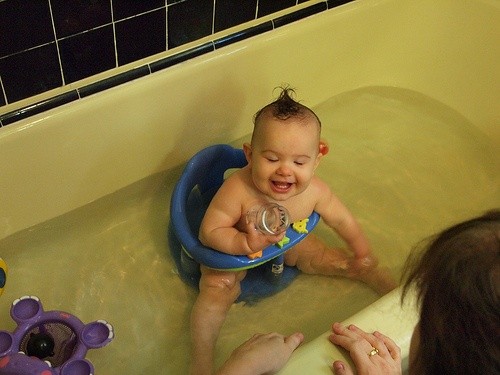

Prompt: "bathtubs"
[1,2,499,372]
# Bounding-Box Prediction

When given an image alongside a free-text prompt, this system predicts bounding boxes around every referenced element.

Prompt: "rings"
[369,348,378,356]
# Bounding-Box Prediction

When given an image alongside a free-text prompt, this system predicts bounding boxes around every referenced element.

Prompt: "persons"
[190,83,399,375]
[215,208,500,375]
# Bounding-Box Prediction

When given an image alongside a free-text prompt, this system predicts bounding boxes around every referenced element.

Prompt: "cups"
[246,200,291,236]
